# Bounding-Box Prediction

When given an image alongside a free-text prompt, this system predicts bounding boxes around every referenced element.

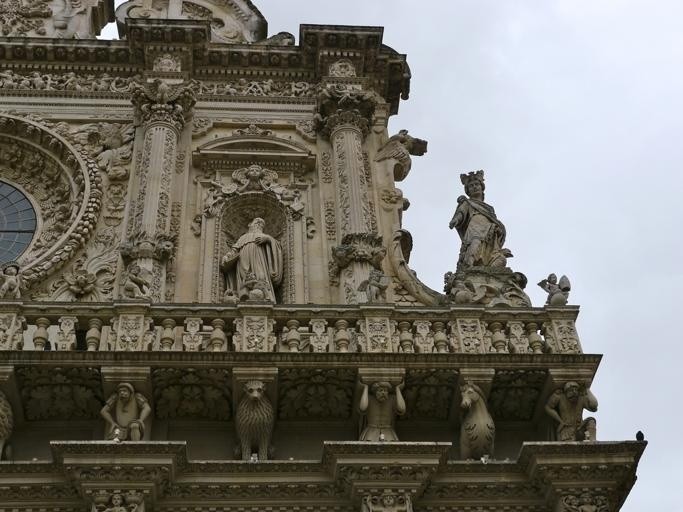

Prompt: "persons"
[98,383,152,441]
[102,491,137,512]
[450,169,515,271]
[124,264,150,298]
[219,216,284,306]
[536,271,571,304]
[355,379,408,443]
[362,491,410,512]
[356,267,389,302]
[561,491,610,512]
[0,263,23,299]
[539,380,598,442]
